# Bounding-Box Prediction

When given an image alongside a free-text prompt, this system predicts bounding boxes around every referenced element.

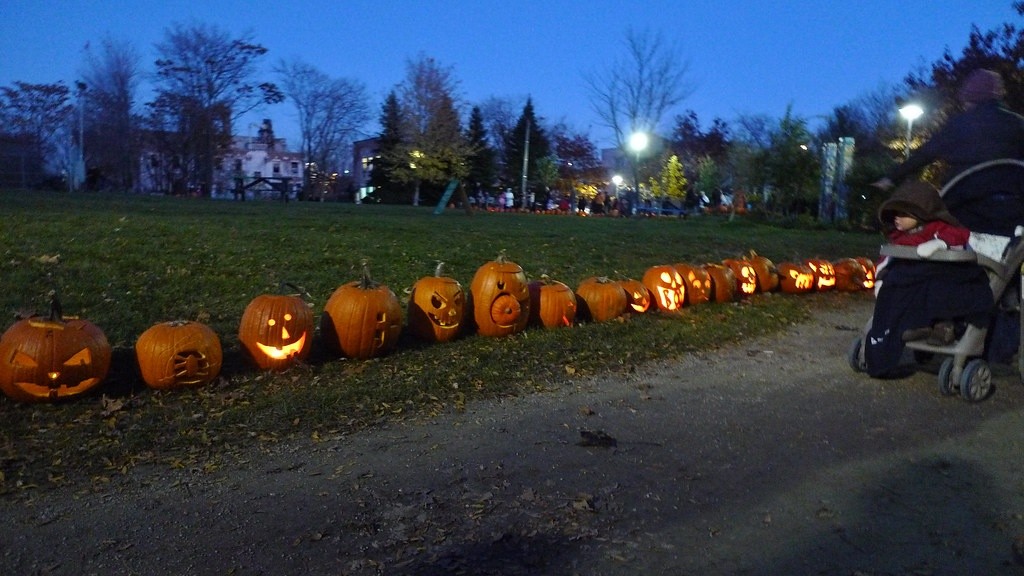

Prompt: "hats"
[881,182,960,227]
[959,69,1005,101]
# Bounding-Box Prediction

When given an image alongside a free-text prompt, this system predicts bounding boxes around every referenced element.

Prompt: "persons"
[469,187,571,211]
[578,196,587,212]
[591,191,629,219]
[871,68,1024,366]
[876,181,971,346]
[699,191,710,216]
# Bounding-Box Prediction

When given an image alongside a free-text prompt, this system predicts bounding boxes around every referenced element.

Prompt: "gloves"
[875,280,883,297]
[917,236,948,258]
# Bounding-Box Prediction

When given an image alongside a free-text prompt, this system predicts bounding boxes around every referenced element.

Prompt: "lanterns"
[321,259,404,359]
[641,249,875,311]
[408,262,465,343]
[0,289,110,401]
[575,276,627,322]
[467,249,530,337]
[527,273,577,330]
[238,282,314,372]
[579,212,587,217]
[616,275,651,314]
[488,207,562,215]
[135,320,222,390]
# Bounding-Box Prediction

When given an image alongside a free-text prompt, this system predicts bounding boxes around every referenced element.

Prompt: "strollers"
[848,158,1024,403]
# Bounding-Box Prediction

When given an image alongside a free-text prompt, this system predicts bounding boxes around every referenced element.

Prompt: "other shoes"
[900,326,933,341]
[928,322,954,344]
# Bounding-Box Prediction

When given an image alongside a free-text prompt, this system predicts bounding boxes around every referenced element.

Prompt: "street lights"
[75,81,88,190]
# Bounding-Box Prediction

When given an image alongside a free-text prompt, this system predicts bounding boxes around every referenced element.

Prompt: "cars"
[633,197,690,220]
